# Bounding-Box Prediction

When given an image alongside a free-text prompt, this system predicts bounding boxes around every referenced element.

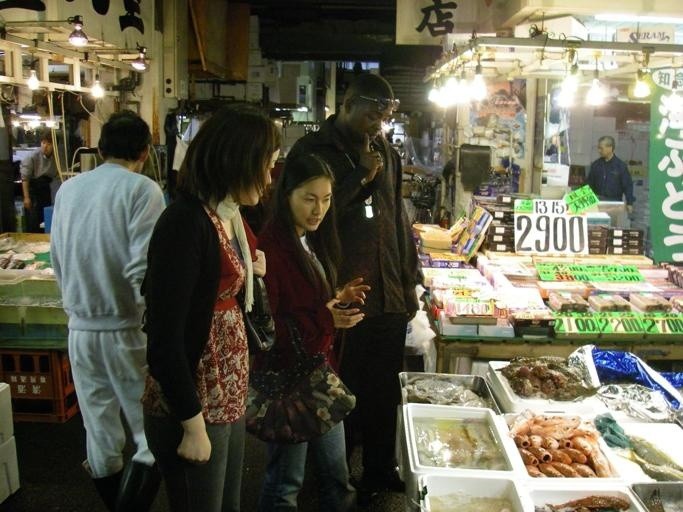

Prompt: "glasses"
[359,94,401,113]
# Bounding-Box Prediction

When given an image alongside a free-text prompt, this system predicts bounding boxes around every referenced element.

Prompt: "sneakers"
[363,468,406,494]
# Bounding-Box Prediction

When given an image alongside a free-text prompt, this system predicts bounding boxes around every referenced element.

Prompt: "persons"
[18,131,59,234]
[547,134,558,156]
[568,136,636,212]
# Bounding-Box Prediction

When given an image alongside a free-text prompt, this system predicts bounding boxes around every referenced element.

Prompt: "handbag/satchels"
[235,273,278,352]
[245,353,358,446]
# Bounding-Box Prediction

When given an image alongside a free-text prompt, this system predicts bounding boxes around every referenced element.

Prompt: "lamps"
[5,15,147,71]
